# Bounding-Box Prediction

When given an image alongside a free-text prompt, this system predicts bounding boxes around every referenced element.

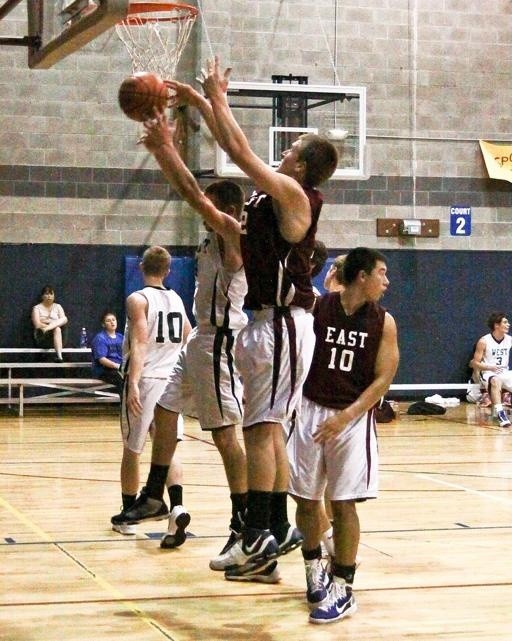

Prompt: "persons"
[163,53,338,570]
[224,240,330,584]
[90,311,124,395]
[470,312,512,427]
[286,246,401,624]
[465,368,511,409]
[30,284,69,363]
[109,105,251,560]
[110,244,195,550]
[322,248,350,568]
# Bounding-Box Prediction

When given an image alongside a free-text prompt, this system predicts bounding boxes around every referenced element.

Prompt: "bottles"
[465,403,487,425]
[392,402,401,420]
[79,328,89,350]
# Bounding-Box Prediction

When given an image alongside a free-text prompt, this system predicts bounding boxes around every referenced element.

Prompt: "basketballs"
[119,73,170,122]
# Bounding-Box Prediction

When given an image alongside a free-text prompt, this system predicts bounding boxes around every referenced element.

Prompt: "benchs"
[1,344,129,417]
[379,381,501,418]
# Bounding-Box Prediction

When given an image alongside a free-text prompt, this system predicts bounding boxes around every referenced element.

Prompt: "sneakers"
[111,487,190,547]
[210,524,305,583]
[305,554,360,623]
[496,410,511,427]
